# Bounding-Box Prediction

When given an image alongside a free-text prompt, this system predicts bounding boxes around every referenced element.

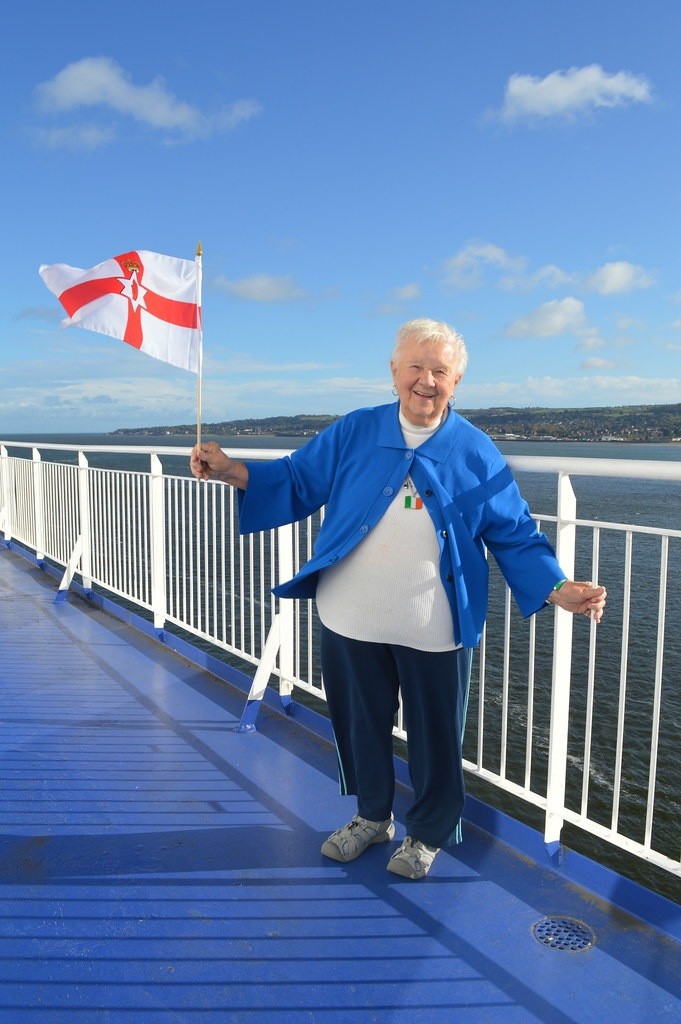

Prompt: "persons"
[191,320,607,881]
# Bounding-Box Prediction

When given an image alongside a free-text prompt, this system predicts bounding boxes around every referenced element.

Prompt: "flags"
[39,249,205,374]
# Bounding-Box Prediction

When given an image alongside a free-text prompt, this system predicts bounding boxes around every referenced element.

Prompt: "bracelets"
[545,579,567,605]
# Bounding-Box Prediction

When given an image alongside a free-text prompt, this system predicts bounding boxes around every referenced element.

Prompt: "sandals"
[388,837,440,879]
[321,810,396,861]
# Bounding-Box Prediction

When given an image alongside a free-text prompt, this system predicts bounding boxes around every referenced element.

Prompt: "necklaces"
[404,474,423,509]
